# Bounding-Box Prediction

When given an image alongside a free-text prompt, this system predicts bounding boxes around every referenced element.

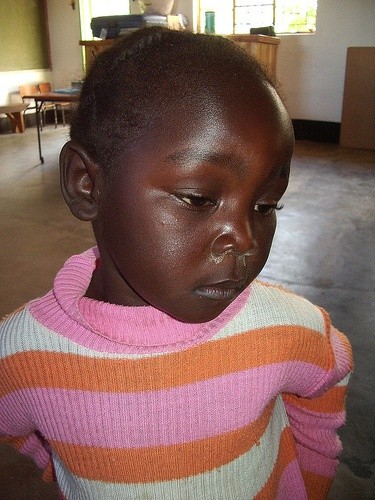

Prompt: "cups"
[205,12,215,34]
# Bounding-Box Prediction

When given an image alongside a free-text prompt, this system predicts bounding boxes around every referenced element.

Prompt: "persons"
[0,26,354,500]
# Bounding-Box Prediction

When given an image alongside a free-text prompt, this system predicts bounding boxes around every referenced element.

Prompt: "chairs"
[19,81,74,132]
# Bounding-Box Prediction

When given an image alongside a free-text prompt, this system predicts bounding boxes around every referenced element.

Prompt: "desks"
[21,92,80,164]
[79,34,281,87]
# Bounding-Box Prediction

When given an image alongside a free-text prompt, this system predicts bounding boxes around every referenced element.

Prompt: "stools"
[0,102,30,133]
